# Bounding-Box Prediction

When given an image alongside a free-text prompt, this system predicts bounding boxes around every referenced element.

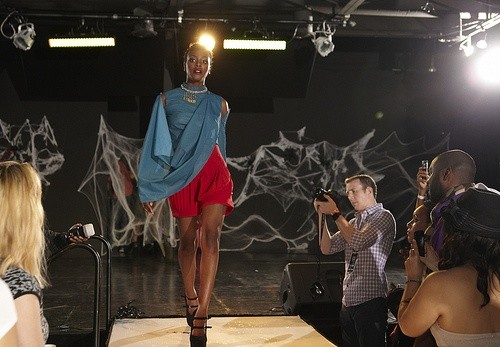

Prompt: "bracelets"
[417,193,425,199]
[331,208,341,221]
[406,280,420,284]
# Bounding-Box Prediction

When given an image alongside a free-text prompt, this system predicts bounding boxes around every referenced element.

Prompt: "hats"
[439,187,500,239]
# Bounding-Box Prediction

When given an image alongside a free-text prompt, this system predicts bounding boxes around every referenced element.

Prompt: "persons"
[416,167,429,208]
[137,43,234,347]
[397,182,500,347]
[427,149,476,273]
[0,161,56,347]
[407,200,437,244]
[43,223,88,257]
[313,174,396,347]
[0,278,20,347]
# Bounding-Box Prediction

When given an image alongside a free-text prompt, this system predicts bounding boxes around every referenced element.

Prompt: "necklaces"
[181,84,208,104]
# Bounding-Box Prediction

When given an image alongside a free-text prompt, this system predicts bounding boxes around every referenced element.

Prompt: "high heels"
[189,309,212,347]
[181,291,199,327]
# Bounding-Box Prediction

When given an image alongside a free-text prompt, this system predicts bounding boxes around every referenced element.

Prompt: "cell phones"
[414,230,426,257]
[422,160,429,173]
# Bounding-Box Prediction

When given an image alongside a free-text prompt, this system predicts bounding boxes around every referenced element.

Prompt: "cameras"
[314,188,338,202]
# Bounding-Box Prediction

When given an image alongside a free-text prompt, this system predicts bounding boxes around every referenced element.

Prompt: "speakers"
[278,262,345,316]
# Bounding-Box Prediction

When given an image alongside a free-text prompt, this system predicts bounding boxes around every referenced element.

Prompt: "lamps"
[313,30,335,57]
[13,23,36,51]
